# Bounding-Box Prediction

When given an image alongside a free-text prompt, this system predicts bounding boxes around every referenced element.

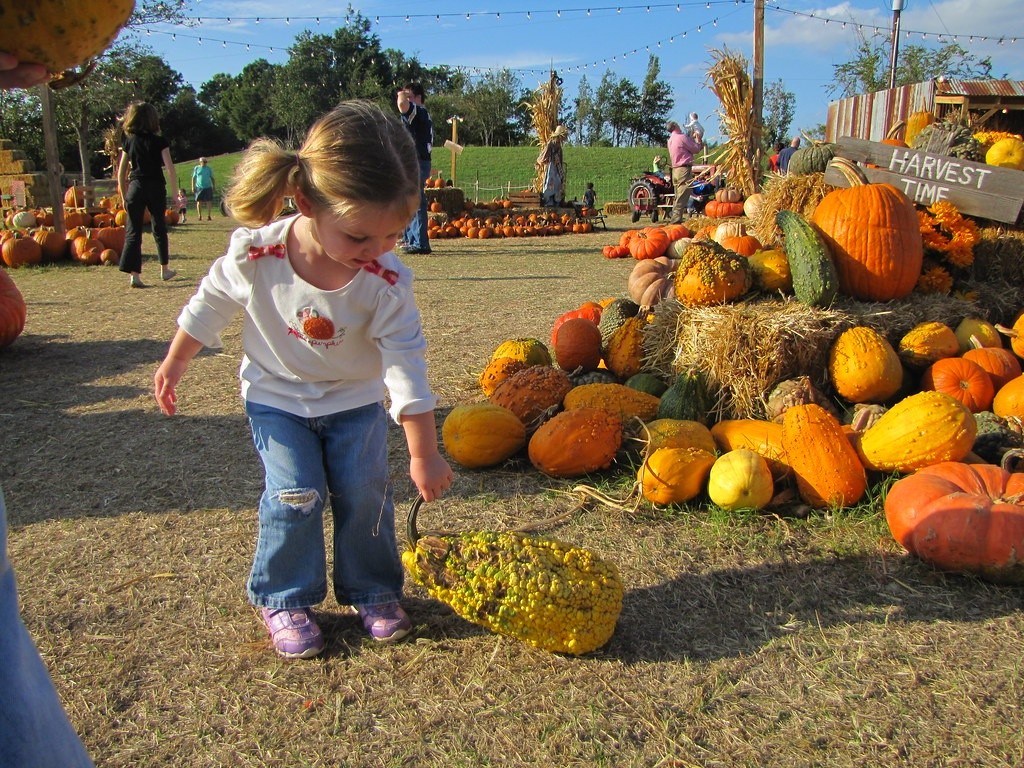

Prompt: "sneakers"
[352,602,412,642]
[396,239,410,248]
[262,607,326,657]
[401,244,431,254]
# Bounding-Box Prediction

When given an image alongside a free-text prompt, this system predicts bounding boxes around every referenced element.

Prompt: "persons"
[534,126,567,207]
[118,102,179,288]
[767,136,800,176]
[582,182,598,211]
[397,82,434,255]
[177,189,188,223]
[0,56,104,768]
[666,112,704,225]
[154,99,455,658]
[653,156,665,179]
[192,157,215,221]
[687,179,714,220]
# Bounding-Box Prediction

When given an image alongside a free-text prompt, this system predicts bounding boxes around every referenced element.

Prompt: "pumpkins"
[0,178,179,349]
[0,0,137,74]
[400,97,1024,656]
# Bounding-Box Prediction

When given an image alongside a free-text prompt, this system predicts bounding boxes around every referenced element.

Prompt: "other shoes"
[130,277,145,288]
[161,268,177,281]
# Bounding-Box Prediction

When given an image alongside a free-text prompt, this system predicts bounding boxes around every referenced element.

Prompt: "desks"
[662,194,675,220]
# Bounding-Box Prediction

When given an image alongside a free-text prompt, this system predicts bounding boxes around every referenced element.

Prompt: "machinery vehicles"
[628,145,727,215]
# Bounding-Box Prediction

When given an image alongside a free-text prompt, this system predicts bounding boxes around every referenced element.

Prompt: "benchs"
[658,205,674,208]
[571,200,608,234]
